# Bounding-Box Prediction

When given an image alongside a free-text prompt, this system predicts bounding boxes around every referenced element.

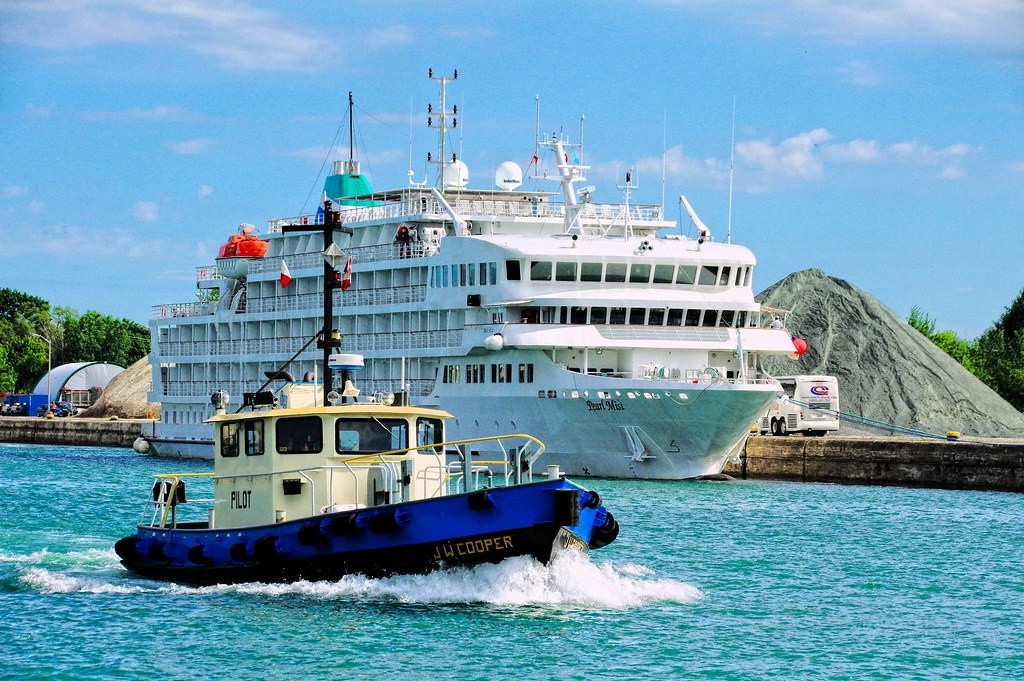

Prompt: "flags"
[564,153,568,164]
[531,154,538,165]
[341,256,351,292]
[572,153,579,165]
[280,260,291,289]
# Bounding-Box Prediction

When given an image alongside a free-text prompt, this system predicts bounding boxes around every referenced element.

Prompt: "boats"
[114,190,623,586]
[136,63,799,480]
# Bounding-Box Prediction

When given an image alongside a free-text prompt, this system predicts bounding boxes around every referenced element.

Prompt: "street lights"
[30,332,51,412]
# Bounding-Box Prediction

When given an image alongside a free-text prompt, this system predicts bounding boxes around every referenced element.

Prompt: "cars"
[51,401,78,417]
[36,404,63,417]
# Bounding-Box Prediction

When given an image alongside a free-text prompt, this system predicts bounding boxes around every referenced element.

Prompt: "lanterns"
[788,339,806,362]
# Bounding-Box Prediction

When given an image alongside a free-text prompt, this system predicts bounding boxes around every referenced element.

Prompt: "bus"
[749,375,840,437]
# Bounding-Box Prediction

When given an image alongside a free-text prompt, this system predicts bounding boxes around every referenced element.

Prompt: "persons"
[398,226,410,260]
